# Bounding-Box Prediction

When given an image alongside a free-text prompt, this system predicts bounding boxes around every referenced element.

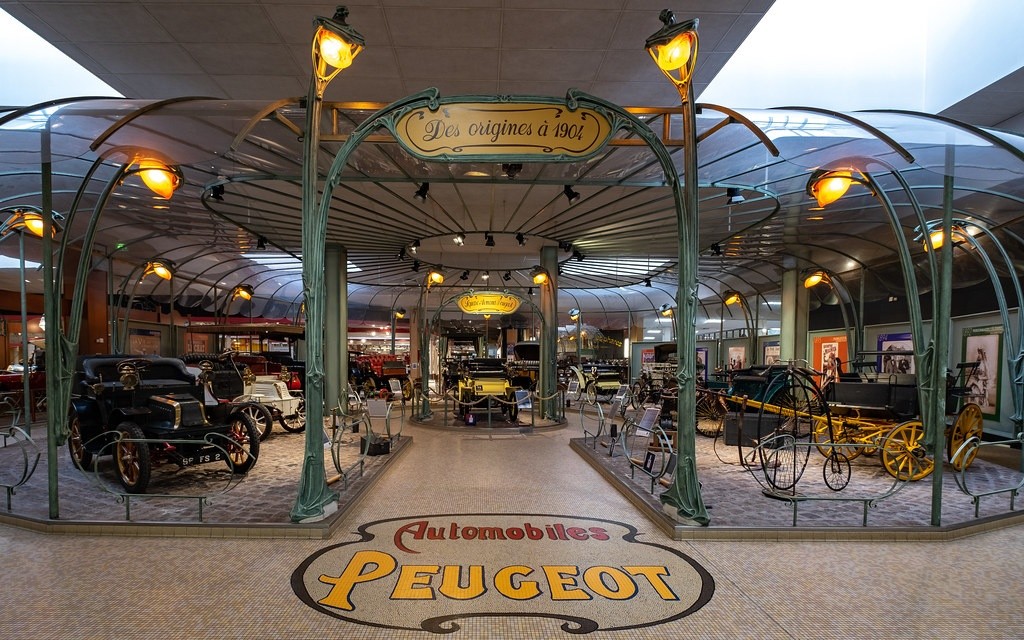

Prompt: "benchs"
[180,353,248,379]
[83,357,198,394]
[834,382,916,408]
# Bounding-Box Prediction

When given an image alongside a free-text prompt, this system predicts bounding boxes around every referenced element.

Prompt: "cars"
[0,370,47,409]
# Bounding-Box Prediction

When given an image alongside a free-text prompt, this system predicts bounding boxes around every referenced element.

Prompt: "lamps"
[113,140,184,201]
[256,236,268,250]
[393,307,407,319]
[799,266,831,290]
[719,290,741,306]
[231,284,254,302]
[567,308,582,321]
[412,182,430,202]
[658,302,674,317]
[801,166,876,210]
[726,187,746,205]
[301,4,371,95]
[560,184,580,205]
[637,7,701,100]
[643,277,652,288]
[396,230,586,286]
[501,162,523,181]
[709,243,723,258]
[3,202,66,241]
[140,255,176,282]
[526,287,533,296]
[208,183,227,203]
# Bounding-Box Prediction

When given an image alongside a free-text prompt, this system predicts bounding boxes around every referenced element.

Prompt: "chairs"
[853,362,881,373]
[949,362,982,393]
[836,357,863,383]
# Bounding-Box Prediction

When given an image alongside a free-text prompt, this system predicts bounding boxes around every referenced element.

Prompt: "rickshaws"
[571,367,621,405]
[696,358,984,482]
[696,365,796,438]
